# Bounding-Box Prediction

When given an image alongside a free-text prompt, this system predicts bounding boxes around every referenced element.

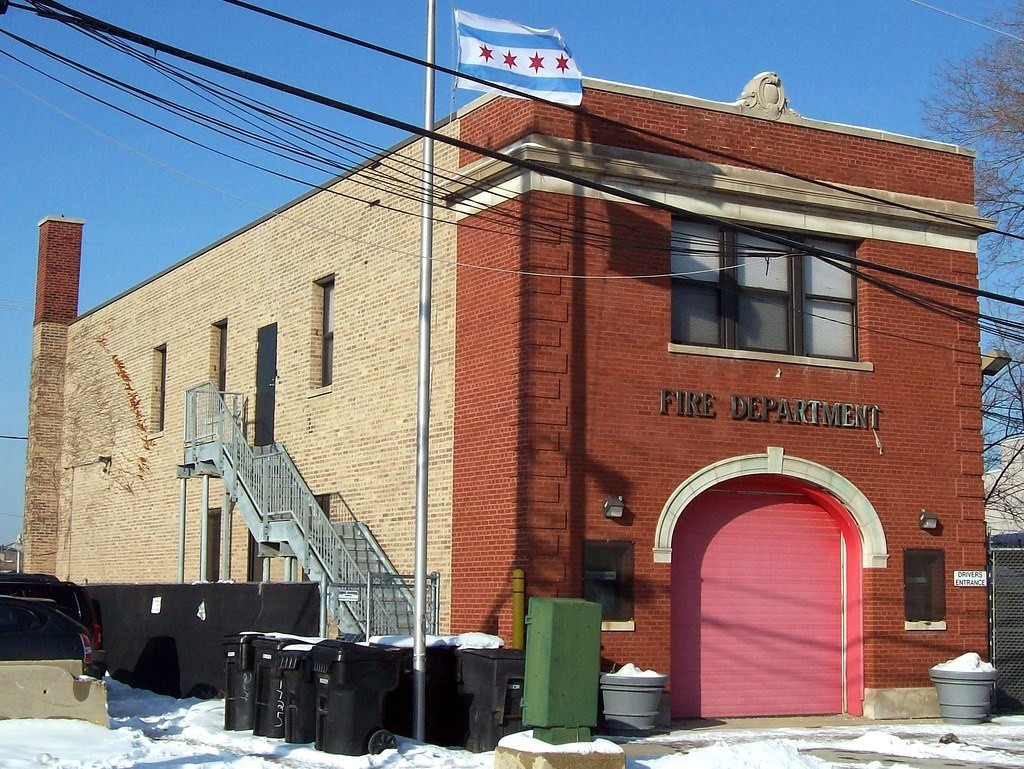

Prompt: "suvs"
[0,594,100,679]
[0,572,108,663]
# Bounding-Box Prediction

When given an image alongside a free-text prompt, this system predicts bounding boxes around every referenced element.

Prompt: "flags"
[454,6,583,106]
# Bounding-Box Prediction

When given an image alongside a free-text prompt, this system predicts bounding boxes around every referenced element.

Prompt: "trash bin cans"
[221,633,264,732]
[250,637,316,744]
[370,641,458,746]
[312,639,401,757]
[458,649,526,754]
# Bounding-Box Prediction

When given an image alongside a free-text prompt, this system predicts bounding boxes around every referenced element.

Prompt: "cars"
[992,566,1019,583]
